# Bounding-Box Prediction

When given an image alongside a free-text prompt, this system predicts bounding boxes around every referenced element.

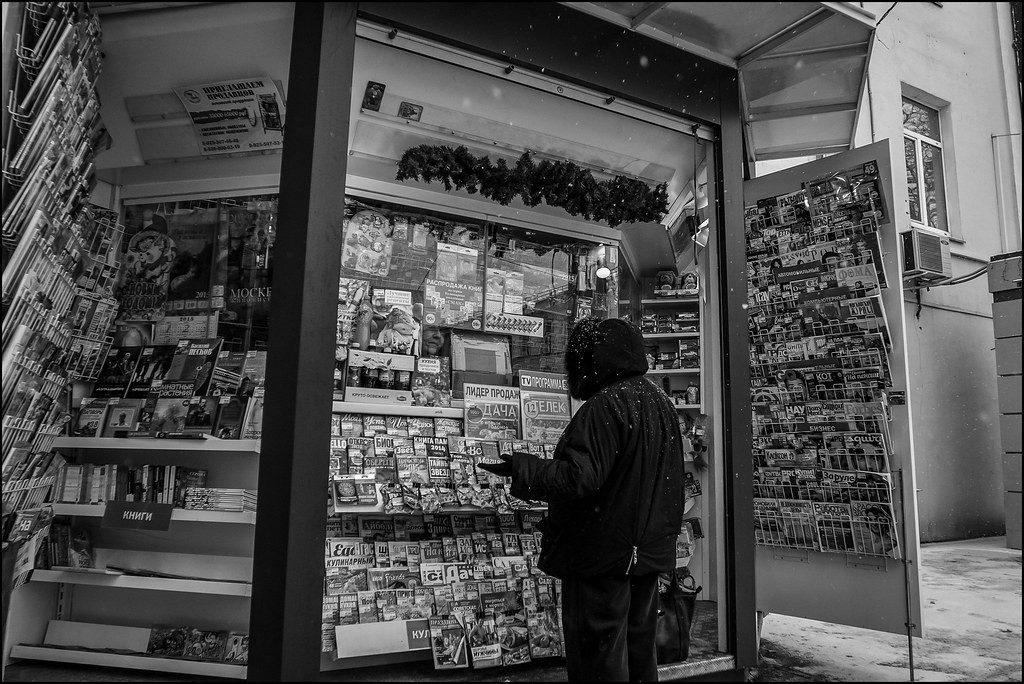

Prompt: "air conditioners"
[901,229,953,282]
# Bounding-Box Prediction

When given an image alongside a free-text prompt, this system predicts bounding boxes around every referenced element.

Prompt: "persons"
[477,317,685,683]
[749,247,899,555]
[422,325,450,357]
[784,370,807,401]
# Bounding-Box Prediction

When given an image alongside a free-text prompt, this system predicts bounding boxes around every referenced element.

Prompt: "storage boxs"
[44,619,153,653]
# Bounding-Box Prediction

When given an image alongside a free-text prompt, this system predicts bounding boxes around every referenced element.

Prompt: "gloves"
[477,454,513,476]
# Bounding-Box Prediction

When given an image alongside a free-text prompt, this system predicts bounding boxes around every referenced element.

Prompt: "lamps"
[596,264,618,278]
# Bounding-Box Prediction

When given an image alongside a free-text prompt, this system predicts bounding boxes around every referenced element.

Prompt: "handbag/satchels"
[655,568,703,665]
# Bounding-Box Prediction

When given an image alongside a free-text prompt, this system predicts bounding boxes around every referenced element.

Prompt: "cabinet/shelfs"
[2,2,736,683]
